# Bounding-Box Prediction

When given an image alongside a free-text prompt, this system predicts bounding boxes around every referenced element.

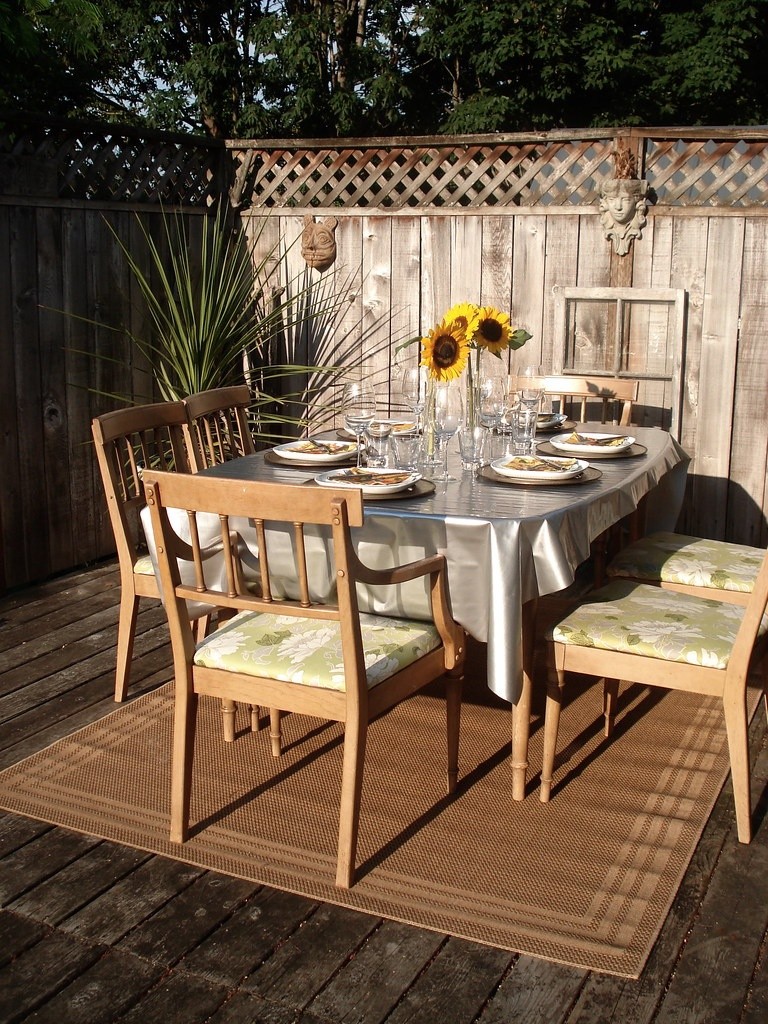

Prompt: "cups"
[363,422,391,468]
[390,433,421,471]
[458,426,488,471]
[510,411,538,456]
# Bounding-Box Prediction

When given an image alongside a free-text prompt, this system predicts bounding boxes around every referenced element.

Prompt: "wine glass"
[515,365,547,456]
[340,381,376,469]
[474,376,508,462]
[427,385,465,484]
[402,366,429,435]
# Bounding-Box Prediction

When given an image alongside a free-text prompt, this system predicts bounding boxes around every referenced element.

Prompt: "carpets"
[0,593,767,980]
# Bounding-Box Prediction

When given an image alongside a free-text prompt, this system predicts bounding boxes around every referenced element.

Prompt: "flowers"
[393,300,534,443]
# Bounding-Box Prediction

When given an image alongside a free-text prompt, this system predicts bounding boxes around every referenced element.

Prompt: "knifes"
[327,472,412,480]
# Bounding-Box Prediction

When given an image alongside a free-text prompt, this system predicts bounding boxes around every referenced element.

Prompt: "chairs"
[143,467,471,888]
[181,384,259,472]
[538,536,768,845]
[507,375,639,578]
[90,400,286,758]
[577,530,767,720]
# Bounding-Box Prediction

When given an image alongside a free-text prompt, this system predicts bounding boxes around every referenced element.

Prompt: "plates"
[314,467,423,495]
[343,419,424,437]
[272,439,369,462]
[549,432,636,454]
[500,412,568,429]
[489,455,590,482]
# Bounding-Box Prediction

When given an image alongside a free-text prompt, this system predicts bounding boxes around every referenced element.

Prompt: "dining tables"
[141,412,692,804]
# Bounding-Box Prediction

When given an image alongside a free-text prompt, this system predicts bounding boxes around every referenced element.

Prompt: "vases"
[457,370,492,470]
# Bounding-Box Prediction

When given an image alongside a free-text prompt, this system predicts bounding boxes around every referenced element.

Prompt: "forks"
[343,469,397,484]
[307,439,338,455]
